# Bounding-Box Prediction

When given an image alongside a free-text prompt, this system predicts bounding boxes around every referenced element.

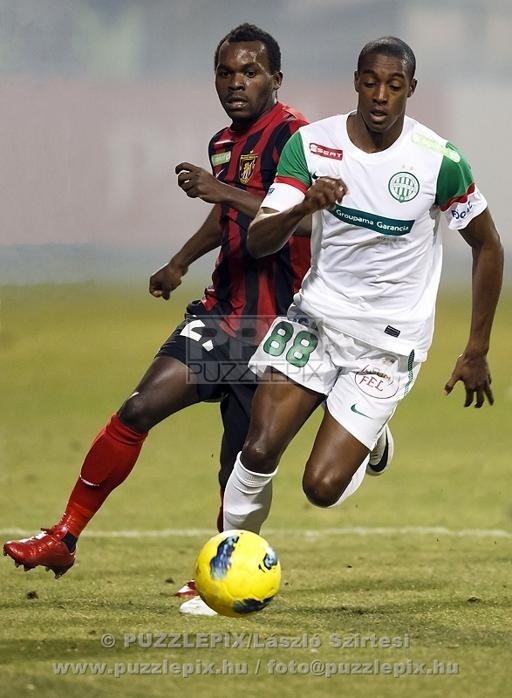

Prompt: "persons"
[177,34,505,622]
[6,25,312,600]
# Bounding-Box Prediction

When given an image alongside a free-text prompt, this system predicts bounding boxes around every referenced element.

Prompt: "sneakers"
[174,578,219,616]
[3,524,79,579]
[366,423,394,477]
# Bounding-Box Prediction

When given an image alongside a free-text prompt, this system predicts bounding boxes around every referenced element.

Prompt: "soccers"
[195,529,281,616]
